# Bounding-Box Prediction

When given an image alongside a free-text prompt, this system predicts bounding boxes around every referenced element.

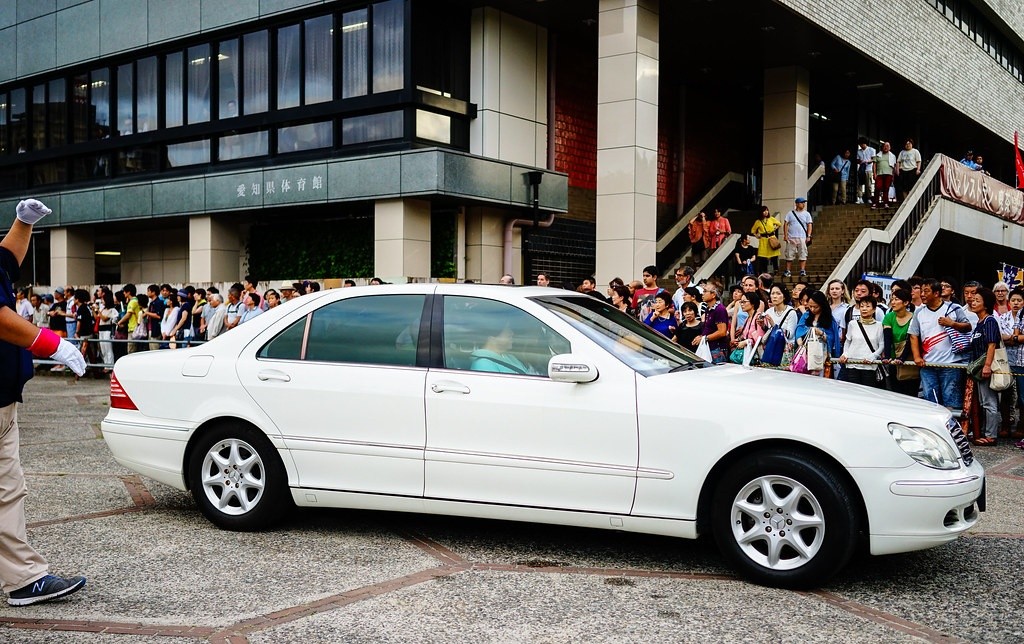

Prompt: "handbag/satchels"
[768,235,781,249]
[131,316,147,340]
[94,316,100,332]
[696,326,827,373]
[874,302,1013,391]
[830,171,841,183]
[806,233,811,246]
[175,324,195,340]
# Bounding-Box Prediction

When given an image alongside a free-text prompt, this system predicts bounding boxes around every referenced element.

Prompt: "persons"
[344,278,393,288]
[0,198,89,608]
[469,309,542,376]
[576,264,728,361]
[511,314,557,368]
[500,273,551,289]
[829,139,924,209]
[961,148,990,176]
[689,194,814,277]
[226,100,237,117]
[729,277,1024,447]
[90,274,324,377]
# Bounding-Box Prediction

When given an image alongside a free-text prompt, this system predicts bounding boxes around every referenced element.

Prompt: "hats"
[967,150,974,155]
[41,287,65,299]
[278,280,296,291]
[177,289,188,298]
[794,197,807,202]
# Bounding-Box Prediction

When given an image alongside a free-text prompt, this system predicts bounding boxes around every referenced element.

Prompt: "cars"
[99,284,986,590]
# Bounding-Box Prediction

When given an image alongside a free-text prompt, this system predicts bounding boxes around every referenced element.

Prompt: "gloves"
[16,198,52,225]
[48,336,87,376]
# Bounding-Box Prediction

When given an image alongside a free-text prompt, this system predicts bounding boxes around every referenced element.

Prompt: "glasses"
[939,284,1008,292]
[610,274,750,302]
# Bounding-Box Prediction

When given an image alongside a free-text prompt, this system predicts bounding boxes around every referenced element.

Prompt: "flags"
[1013,134,1024,190]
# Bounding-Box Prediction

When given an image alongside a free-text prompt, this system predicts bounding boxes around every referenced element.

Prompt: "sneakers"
[831,197,891,209]
[775,270,807,277]
[974,429,1024,447]
[52,365,113,373]
[7,575,86,606]
